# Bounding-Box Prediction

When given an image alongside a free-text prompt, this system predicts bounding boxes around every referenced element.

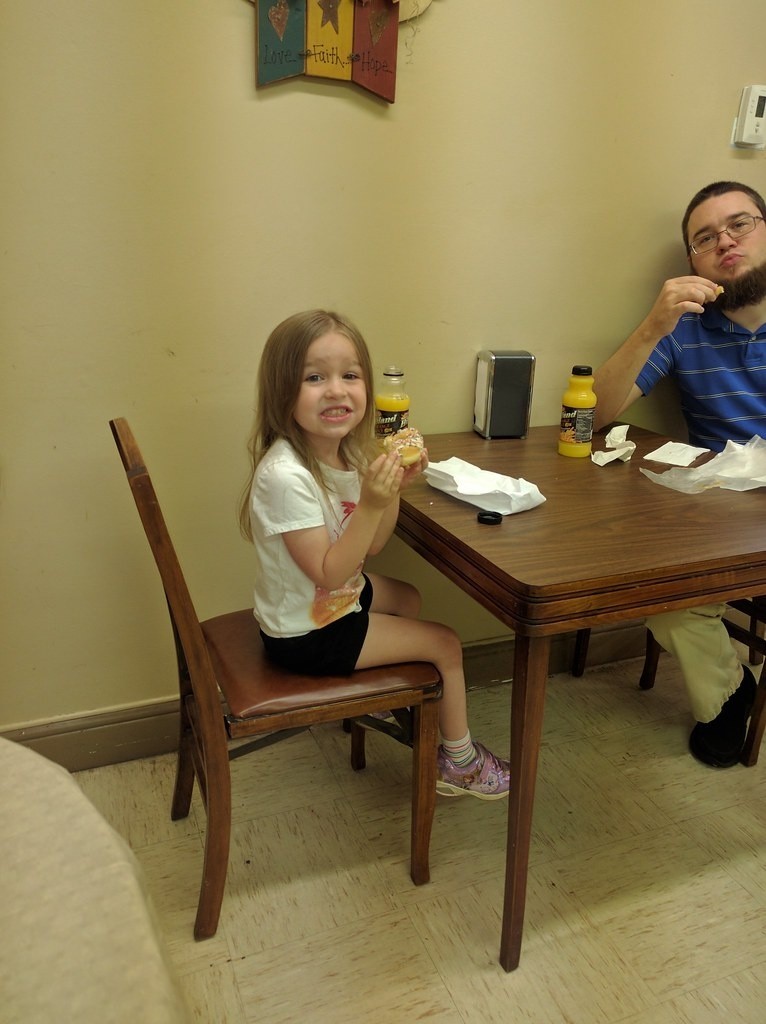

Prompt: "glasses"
[687,215,764,255]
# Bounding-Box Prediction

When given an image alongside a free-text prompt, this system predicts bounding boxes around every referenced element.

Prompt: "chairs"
[638,596,766,768]
[108,416,446,942]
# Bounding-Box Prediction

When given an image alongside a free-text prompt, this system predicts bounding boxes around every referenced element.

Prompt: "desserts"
[383,427,424,467]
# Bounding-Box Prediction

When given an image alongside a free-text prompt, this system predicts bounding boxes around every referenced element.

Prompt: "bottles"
[373,366,411,447]
[557,365,597,458]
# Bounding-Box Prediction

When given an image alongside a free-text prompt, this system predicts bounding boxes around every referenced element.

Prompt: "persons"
[591,180,766,770]
[234,309,511,801]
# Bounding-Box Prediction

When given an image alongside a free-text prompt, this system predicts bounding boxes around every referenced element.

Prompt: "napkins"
[643,440,711,467]
[590,425,636,466]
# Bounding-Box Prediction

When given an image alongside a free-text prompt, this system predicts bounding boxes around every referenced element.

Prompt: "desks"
[357,424,766,972]
[0,736,189,1024]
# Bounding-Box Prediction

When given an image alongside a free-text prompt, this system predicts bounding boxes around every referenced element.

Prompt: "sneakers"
[368,706,395,723]
[435,741,511,800]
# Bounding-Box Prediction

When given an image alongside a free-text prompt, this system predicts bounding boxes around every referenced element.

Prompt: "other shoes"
[689,664,757,768]
